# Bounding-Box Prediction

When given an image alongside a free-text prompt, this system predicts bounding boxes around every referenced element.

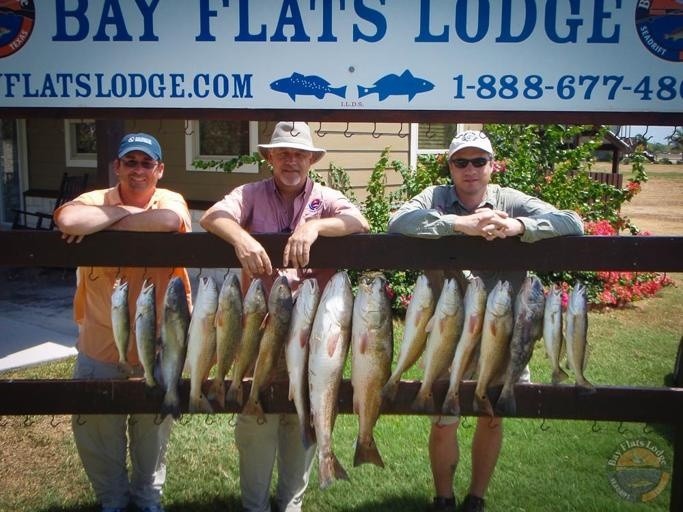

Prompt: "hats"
[257,121,328,165]
[117,133,163,161]
[448,130,494,161]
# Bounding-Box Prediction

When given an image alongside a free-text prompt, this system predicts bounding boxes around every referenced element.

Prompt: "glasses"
[451,158,491,169]
[119,158,160,170]
[280,227,294,233]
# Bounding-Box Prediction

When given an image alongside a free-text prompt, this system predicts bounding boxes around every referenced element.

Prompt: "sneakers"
[431,493,456,512]
[456,494,485,511]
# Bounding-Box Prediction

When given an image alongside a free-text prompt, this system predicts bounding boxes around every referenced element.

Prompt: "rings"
[485,232,495,238]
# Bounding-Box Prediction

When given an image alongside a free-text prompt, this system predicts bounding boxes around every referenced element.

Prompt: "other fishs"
[134,277,162,401]
[155,274,291,426]
[357,71,436,101]
[110,277,132,374]
[384,271,596,419]
[269,71,348,102]
[283,270,394,486]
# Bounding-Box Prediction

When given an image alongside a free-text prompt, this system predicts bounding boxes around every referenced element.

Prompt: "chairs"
[10,172,87,229]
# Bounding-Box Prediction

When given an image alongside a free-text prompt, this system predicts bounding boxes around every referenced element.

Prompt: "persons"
[385,129,585,512]
[52,132,193,512]
[199,119,372,512]
